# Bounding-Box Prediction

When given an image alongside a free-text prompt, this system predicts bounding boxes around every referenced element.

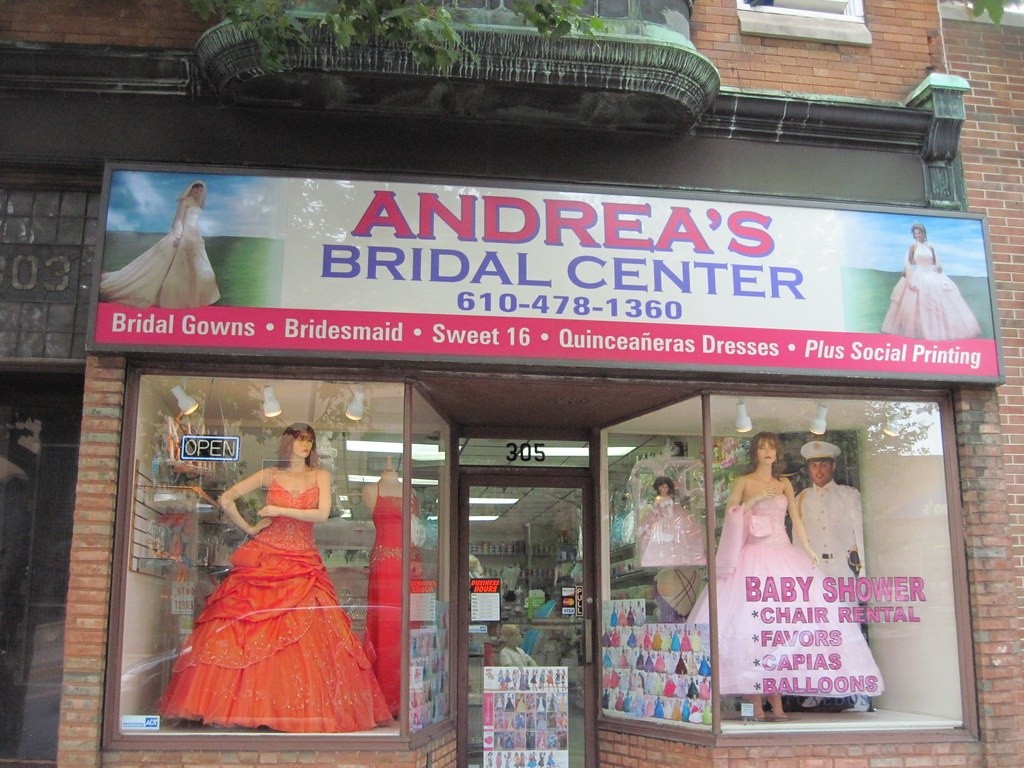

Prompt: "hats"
[799,442,841,462]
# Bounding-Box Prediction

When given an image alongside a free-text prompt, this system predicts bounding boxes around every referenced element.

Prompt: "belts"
[813,553,851,560]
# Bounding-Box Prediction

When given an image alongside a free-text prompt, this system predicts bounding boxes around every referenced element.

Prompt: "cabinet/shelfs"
[474,552,554,580]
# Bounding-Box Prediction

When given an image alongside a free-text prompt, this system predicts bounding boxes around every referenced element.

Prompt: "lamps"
[807,404,828,435]
[170,384,199,417]
[262,385,282,418]
[735,397,753,433]
[345,392,365,421]
[883,410,909,436]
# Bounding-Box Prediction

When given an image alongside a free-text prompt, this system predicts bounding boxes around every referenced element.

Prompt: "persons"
[98,171,224,312]
[779,438,877,714]
[715,429,887,722]
[635,474,710,564]
[881,223,984,342]
[165,422,400,733]
[502,624,540,669]
